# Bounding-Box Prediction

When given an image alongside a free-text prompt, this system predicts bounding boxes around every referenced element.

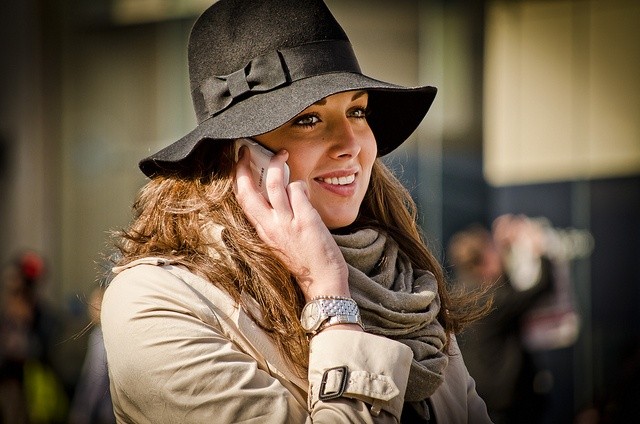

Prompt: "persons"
[97,0,492,424]
[445,211,592,424]
[0,250,57,423]
[70,284,116,424]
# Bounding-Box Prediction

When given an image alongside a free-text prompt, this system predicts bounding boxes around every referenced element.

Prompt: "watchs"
[300,299,365,341]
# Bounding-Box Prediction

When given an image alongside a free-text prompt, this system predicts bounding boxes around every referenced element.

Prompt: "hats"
[138,0,438,184]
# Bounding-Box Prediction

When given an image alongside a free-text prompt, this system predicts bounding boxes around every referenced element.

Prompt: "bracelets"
[306,295,357,303]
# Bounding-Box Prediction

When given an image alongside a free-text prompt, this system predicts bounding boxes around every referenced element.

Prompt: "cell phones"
[232,139,291,203]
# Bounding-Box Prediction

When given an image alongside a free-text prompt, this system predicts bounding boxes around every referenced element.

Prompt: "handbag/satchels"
[24,364,65,422]
